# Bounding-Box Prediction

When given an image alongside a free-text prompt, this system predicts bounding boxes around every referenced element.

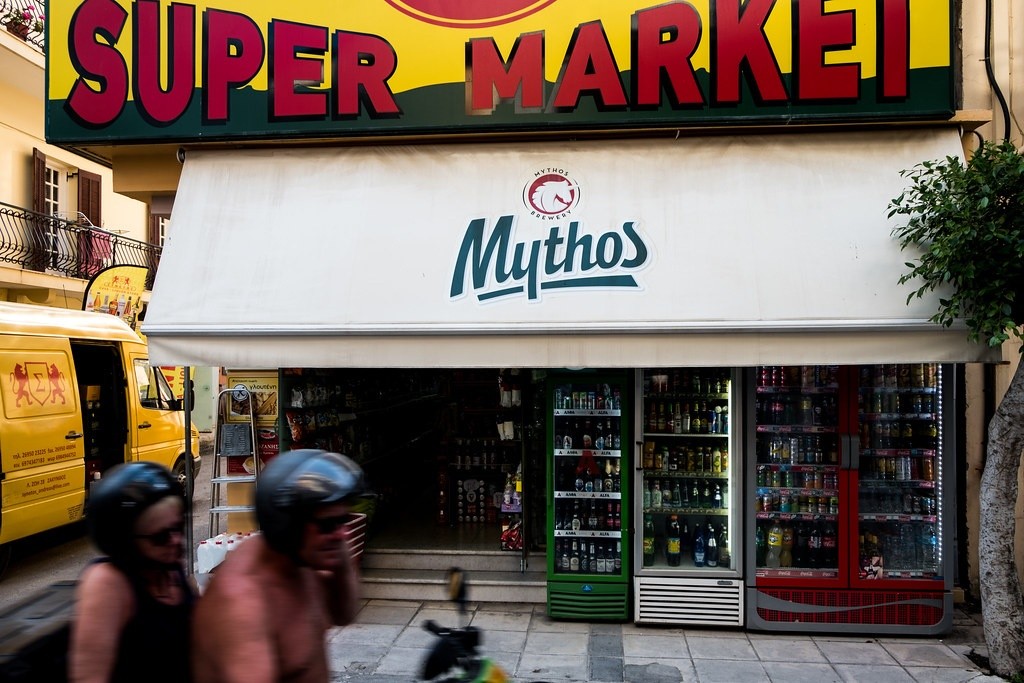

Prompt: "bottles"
[456,439,509,473]
[756,363,938,577]
[502,514,511,533]
[503,482,513,504]
[644,368,731,568]
[553,379,622,574]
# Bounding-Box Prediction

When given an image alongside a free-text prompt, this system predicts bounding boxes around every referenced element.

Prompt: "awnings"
[144,122,1009,368]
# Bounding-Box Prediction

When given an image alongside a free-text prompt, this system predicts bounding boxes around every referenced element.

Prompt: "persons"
[190,448,361,683]
[69,461,202,683]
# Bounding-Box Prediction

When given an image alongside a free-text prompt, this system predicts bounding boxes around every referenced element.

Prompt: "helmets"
[254,449,363,555]
[85,462,184,565]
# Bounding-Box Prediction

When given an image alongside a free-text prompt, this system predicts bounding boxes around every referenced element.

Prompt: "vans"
[0,298,203,578]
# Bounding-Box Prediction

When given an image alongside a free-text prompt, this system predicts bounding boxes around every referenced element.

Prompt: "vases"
[7,21,31,42]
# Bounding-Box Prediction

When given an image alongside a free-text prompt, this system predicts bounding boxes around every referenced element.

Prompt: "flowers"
[3,5,45,31]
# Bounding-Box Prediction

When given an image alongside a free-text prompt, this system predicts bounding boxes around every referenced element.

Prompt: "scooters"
[414,562,507,683]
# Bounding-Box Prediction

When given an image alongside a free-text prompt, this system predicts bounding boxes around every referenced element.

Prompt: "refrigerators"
[634,368,744,626]
[545,368,630,619]
[746,363,956,636]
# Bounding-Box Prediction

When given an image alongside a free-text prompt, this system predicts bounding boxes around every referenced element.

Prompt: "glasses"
[311,511,352,534]
[136,516,184,547]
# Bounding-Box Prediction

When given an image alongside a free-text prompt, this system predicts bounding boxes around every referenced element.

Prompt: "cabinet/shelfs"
[279,369,523,551]
